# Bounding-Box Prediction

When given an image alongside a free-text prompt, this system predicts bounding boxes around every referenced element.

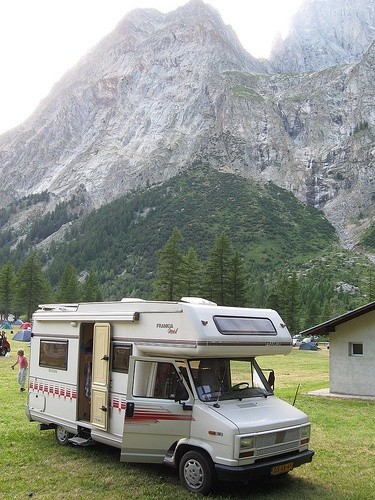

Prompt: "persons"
[11,349,28,391]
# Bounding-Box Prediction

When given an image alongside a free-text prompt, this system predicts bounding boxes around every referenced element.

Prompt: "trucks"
[26,296,314,493]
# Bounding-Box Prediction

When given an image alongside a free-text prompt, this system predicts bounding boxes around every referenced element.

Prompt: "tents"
[11,329,31,341]
[0,322,13,329]
[20,322,32,329]
[13,319,24,325]
[292,335,321,351]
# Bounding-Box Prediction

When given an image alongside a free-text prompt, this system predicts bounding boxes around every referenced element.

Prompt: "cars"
[292,339,302,347]
[302,338,312,344]
[0,331,10,356]
